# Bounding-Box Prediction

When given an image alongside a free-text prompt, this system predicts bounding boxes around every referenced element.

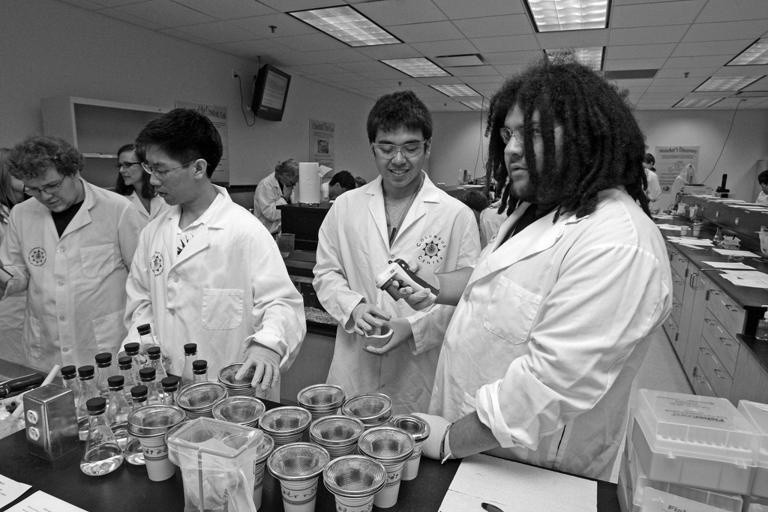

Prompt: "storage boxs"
[618,388,768,512]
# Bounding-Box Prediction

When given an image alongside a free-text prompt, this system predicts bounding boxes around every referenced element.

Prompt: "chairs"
[229,191,255,214]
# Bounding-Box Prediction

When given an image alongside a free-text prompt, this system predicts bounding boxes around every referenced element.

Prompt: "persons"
[410,48,674,483]
[463,188,508,249]
[310,89,482,418]
[636,153,661,207]
[354,176,367,189]
[0,130,147,382]
[0,145,30,365]
[114,142,169,223]
[320,169,357,204]
[252,157,299,241]
[755,169,768,206]
[116,106,307,406]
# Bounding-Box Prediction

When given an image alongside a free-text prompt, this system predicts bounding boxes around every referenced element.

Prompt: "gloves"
[235,342,281,392]
[352,302,392,335]
[363,318,413,356]
[412,412,456,465]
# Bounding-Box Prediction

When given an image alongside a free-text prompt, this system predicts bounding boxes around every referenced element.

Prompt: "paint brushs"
[482,503,504,512]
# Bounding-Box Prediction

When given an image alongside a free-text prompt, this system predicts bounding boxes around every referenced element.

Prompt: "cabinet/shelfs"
[41,96,174,190]
[276,177,497,338]
[647,192,767,407]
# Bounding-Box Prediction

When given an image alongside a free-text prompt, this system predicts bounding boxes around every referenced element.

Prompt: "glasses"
[116,161,138,169]
[500,126,540,144]
[23,175,65,196]
[372,139,426,159]
[141,160,192,178]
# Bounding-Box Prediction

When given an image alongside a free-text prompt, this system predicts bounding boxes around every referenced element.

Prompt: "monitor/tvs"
[250,63,291,122]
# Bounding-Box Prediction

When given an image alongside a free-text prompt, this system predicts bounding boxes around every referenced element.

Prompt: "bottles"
[713,227,722,245]
[60,323,209,476]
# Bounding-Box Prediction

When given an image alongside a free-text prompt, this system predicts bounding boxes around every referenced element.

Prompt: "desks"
[0,357,616,512]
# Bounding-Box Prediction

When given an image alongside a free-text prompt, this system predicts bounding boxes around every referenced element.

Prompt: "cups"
[126,404,187,481]
[692,223,701,237]
[361,325,394,348]
[321,454,389,512]
[276,233,295,259]
[267,442,330,512]
[178,382,226,419]
[255,405,313,445]
[340,391,393,427]
[680,226,688,236]
[252,431,274,512]
[295,383,346,419]
[359,427,415,509]
[387,414,431,482]
[218,365,258,397]
[210,395,266,429]
